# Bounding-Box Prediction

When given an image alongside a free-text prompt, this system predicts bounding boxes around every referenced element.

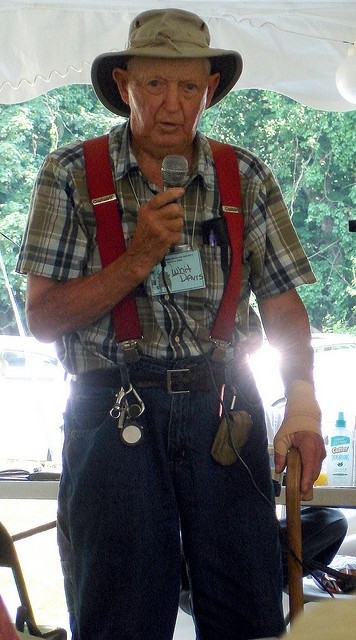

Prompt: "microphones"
[161,157,190,193]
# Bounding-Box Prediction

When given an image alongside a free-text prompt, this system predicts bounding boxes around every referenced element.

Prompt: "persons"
[13,7,326,639]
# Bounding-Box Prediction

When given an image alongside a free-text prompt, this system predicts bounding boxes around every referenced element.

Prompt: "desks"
[0,480,355,510]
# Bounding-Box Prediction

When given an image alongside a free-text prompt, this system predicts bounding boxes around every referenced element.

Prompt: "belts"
[72,365,251,395]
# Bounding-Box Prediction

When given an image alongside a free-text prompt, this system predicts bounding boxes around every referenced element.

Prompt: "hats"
[91,9,243,118]
